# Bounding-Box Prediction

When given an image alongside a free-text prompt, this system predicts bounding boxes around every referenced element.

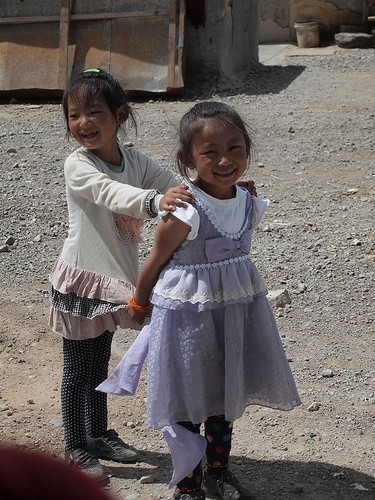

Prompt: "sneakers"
[65,446,108,482]
[86,429,139,461]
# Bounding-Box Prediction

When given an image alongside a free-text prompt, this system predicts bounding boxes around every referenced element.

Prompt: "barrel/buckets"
[294,21,320,47]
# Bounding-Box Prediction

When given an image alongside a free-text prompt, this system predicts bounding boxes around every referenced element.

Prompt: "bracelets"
[129,297,153,313]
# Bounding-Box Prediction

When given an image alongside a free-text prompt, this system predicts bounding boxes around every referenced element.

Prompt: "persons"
[48,68,257,482]
[95,102,302,500]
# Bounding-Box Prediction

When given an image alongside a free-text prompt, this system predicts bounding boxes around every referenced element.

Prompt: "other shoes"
[203,466,251,500]
[173,489,205,500]
